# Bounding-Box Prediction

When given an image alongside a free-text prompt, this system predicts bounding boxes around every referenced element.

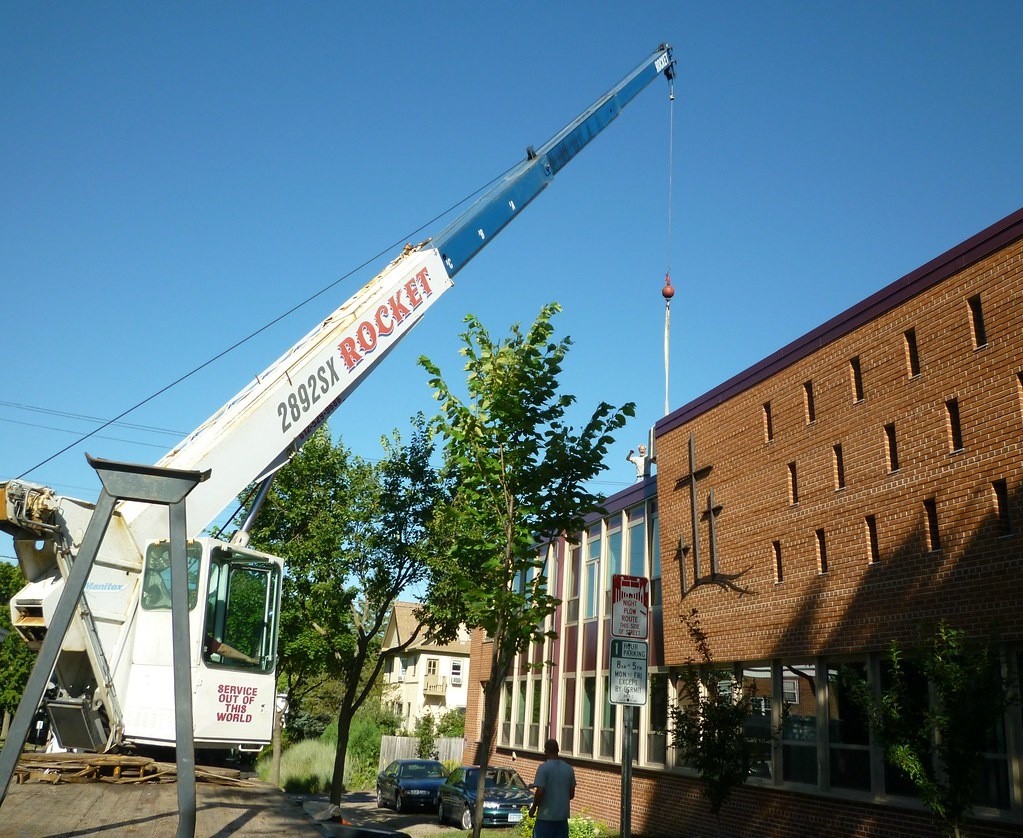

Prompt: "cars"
[434,764,536,831]
[376,757,451,814]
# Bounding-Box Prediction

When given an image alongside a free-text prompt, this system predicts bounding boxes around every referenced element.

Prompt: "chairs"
[425,766,434,774]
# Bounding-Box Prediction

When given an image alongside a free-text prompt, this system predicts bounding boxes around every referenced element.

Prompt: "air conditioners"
[398,675,404,682]
[452,677,463,684]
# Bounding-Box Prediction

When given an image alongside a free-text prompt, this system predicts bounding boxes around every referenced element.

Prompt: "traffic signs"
[609,639,648,708]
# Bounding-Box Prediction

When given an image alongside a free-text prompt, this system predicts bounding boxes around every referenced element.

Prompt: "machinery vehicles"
[0,40,682,772]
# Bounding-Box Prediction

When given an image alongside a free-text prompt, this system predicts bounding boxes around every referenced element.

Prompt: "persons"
[529,739,577,838]
[190,598,260,666]
